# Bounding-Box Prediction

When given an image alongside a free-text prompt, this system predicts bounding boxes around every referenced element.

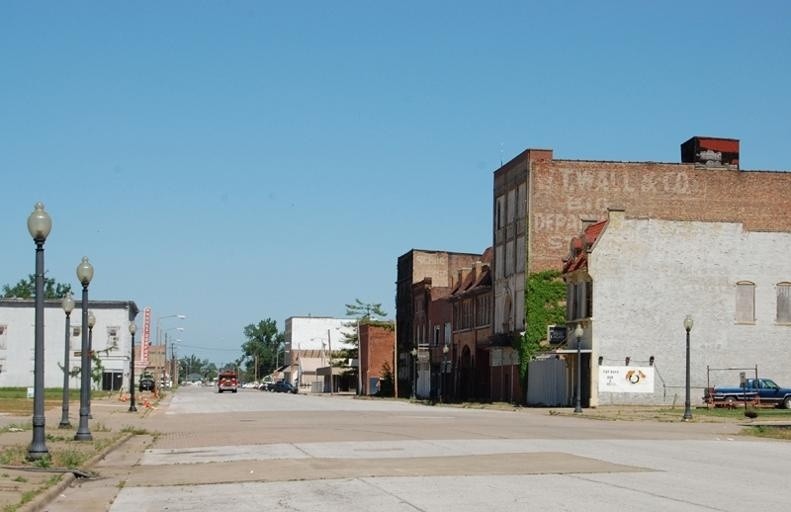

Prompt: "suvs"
[139,379,155,393]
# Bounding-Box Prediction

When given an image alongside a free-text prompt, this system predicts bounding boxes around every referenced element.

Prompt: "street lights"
[152,309,187,401]
[310,335,335,397]
[411,347,418,400]
[574,324,583,413]
[441,343,450,405]
[22,203,140,460]
[682,313,694,418]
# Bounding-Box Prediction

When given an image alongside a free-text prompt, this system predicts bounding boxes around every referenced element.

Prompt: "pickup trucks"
[266,380,299,393]
[703,376,791,411]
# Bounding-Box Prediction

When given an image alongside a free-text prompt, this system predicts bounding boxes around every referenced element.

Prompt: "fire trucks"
[217,373,238,393]
